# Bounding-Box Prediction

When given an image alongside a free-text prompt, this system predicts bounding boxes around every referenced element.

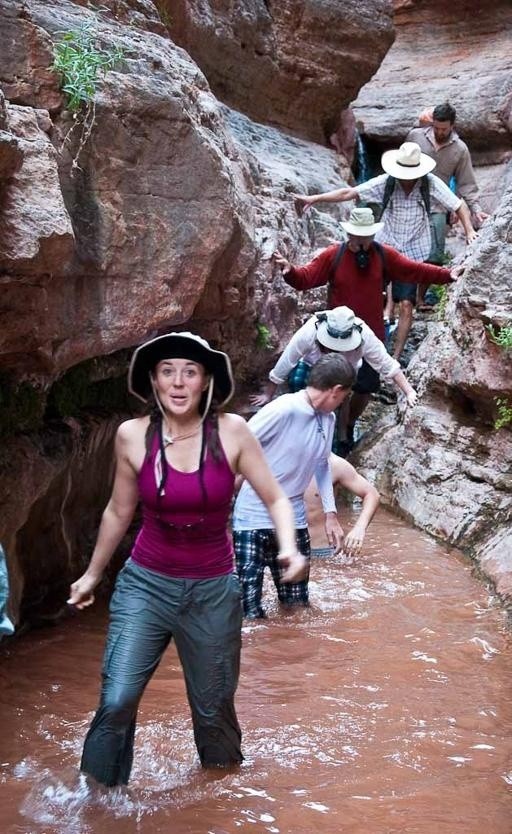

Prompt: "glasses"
[348,234,374,239]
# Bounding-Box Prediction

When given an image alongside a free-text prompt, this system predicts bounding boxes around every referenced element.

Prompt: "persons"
[247,305,417,457]
[399,101,490,310]
[233,353,380,621]
[289,142,481,319]
[273,209,466,452]
[66,332,309,788]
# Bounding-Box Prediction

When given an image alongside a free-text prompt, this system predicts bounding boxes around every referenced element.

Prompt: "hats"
[413,106,436,128]
[339,208,385,236]
[128,332,236,410]
[381,142,436,180]
[317,305,361,352]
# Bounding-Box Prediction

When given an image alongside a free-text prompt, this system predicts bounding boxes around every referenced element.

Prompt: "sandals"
[348,424,354,440]
[384,315,395,326]
[337,439,349,445]
[416,303,433,312]
